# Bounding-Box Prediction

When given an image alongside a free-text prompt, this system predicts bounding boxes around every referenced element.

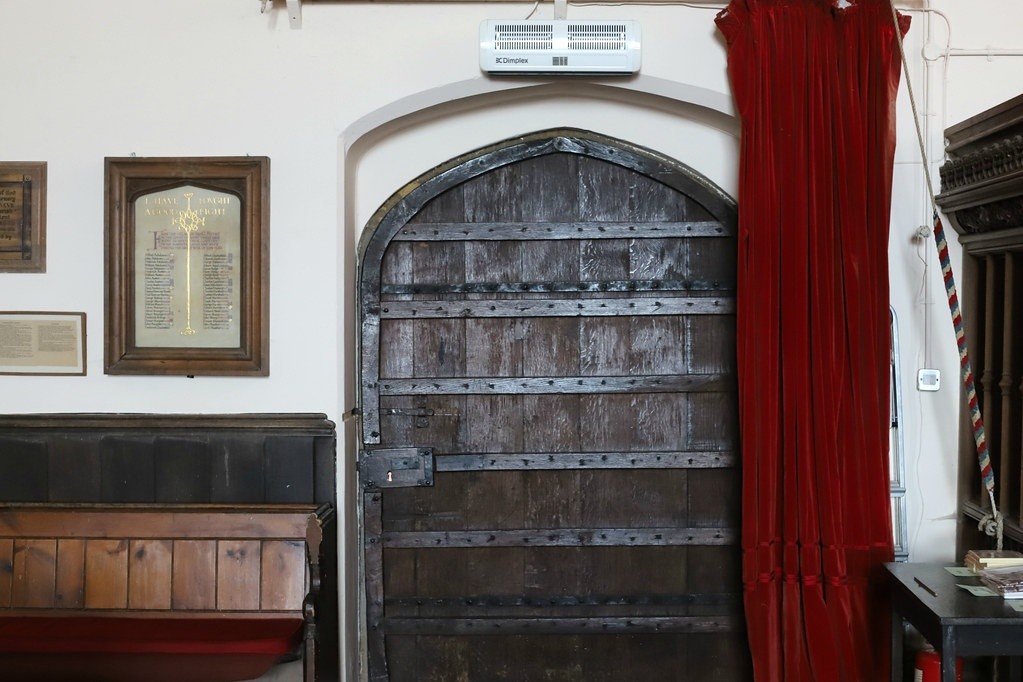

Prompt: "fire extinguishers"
[914,640,963,682]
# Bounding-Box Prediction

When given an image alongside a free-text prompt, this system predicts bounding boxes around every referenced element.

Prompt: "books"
[964,548,1023,601]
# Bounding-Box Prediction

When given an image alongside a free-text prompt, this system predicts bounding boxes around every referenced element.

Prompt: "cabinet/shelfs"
[935,93,1023,560]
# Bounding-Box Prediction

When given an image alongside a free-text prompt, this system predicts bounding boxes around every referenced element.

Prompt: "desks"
[882,561,1023,682]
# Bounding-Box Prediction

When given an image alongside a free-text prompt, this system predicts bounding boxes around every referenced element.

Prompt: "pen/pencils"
[914,577,937,596]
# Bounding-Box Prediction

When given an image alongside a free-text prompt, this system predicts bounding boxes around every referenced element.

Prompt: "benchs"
[0,503,335,682]
